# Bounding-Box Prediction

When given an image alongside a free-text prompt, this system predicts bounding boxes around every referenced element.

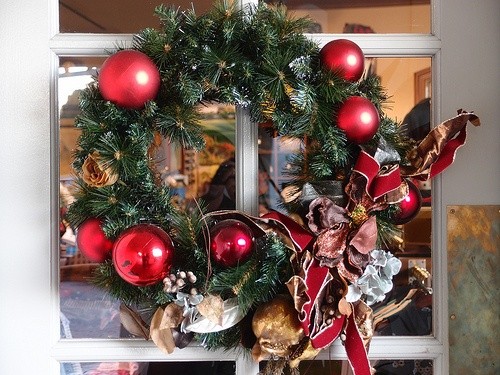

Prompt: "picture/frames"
[414,67,431,105]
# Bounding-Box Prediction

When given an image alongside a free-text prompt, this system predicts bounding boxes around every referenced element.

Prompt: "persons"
[258,156,283,217]
